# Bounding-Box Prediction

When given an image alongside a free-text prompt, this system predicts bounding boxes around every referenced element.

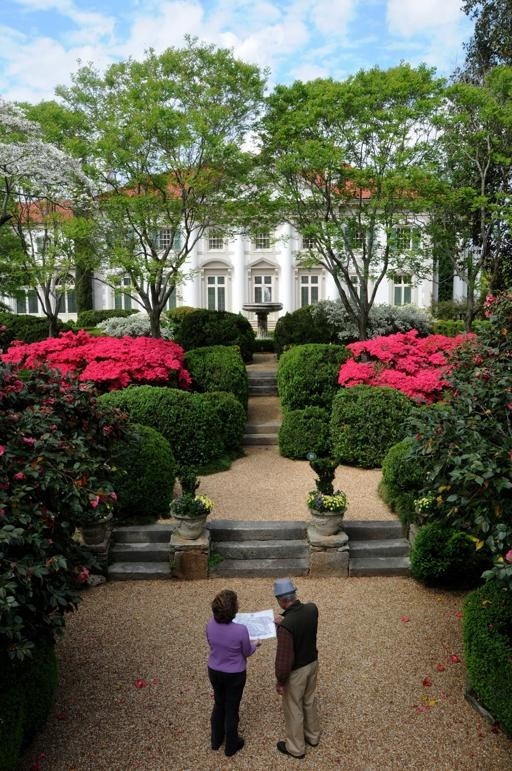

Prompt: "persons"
[202,590,263,756]
[269,578,322,759]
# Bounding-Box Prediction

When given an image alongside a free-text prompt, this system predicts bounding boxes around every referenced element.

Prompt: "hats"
[272,578,296,596]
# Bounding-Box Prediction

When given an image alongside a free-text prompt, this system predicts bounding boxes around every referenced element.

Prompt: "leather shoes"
[305,738,318,747]
[277,742,305,759]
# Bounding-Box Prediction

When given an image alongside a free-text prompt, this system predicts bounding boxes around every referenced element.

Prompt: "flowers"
[170,495,214,515]
[306,491,347,512]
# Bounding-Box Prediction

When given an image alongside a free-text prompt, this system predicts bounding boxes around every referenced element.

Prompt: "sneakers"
[225,739,244,756]
[212,745,219,750]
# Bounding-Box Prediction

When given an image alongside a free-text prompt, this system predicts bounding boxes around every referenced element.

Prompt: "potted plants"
[167,463,214,540]
[304,454,349,536]
